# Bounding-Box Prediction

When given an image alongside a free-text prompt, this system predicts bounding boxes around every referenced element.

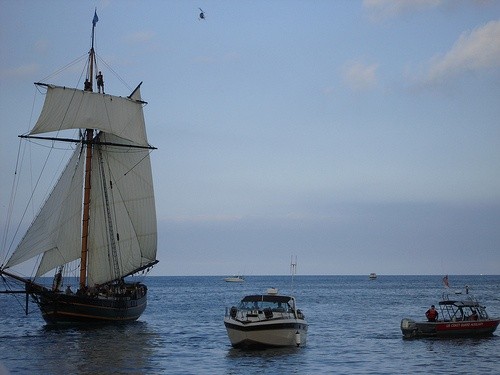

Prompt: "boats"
[400,275,500,339]
[369,273,376,280]
[223,275,246,282]
[222,255,309,350]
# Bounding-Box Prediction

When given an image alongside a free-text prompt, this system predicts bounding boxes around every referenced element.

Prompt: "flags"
[444,274,449,287]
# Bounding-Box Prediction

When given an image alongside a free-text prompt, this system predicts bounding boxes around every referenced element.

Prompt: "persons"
[96,71,105,94]
[230,306,237,318]
[254,302,260,309]
[278,302,285,310]
[425,305,438,322]
[125,287,132,295]
[84,79,93,92]
[55,270,62,291]
[467,310,478,321]
[66,284,107,297]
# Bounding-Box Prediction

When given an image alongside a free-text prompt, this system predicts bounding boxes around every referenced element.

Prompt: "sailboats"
[0,7,159,325]
